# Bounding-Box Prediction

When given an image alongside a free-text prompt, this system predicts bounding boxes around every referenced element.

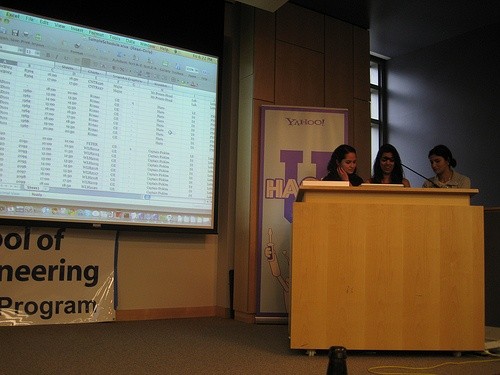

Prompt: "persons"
[321,144,364,186]
[369,143,411,187]
[422,145,471,189]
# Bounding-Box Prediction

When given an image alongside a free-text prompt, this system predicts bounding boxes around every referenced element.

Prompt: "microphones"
[384,156,441,188]
